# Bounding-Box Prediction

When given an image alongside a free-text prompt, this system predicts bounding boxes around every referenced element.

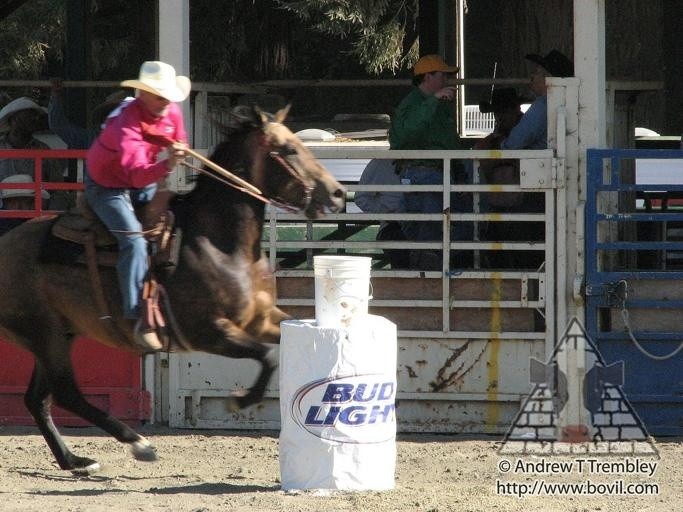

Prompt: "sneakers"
[119,312,162,352]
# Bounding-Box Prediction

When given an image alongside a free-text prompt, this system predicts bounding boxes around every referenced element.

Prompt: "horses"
[0,98,348,475]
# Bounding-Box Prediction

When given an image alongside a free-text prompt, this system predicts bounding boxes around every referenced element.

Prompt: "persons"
[83,59,192,351]
[48,76,135,148]
[473,86,535,150]
[0,96,77,210]
[353,147,410,267]
[387,53,468,270]
[498,48,574,269]
[0,173,51,235]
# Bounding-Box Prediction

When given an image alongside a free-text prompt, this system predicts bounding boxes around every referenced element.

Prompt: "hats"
[414,55,460,75]
[479,88,536,112]
[93,89,131,125]
[526,50,574,77]
[0,97,48,127]
[121,60,191,102]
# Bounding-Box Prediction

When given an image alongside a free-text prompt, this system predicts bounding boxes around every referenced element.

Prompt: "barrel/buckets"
[278,315,397,492]
[312,254,373,330]
